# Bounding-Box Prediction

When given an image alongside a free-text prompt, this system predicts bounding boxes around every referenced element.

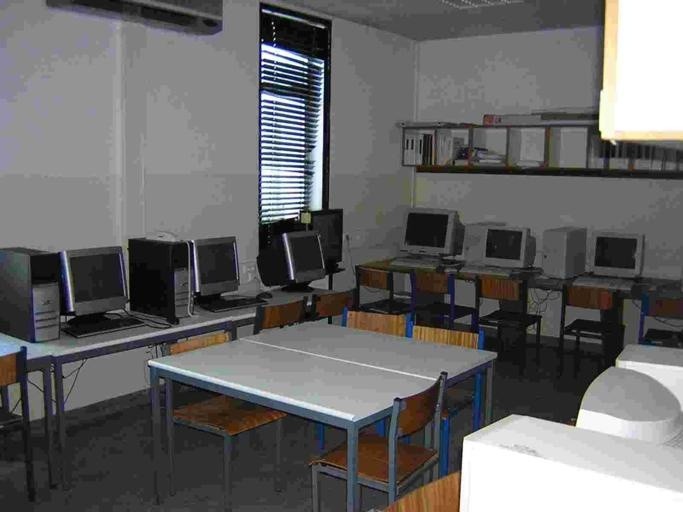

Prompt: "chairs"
[309,287,359,322]
[252,295,308,333]
[637,289,683,349]
[308,369,448,511]
[318,306,412,452]
[383,469,463,512]
[355,265,414,317]
[473,275,542,374]
[0,346,37,501]
[386,322,485,480]
[162,328,290,512]
[409,267,477,332]
[556,281,625,378]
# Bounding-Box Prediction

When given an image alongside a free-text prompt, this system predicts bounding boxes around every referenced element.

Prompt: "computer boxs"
[616,343,683,411]
[0,246,62,343]
[128,237,195,318]
[460,413,683,509]
[542,226,587,280]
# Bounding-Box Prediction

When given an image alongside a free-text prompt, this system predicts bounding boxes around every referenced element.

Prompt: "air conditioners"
[48,0,224,35]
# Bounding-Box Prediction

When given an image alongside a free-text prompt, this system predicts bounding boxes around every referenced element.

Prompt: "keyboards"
[61,316,146,339]
[199,297,268,312]
[388,256,440,270]
[459,264,512,278]
[573,275,636,292]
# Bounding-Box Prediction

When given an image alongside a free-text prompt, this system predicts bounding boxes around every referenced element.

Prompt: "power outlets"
[342,233,352,251]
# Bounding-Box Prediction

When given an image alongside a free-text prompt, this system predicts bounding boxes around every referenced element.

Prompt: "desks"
[195,284,337,340]
[147,339,438,512]
[0,332,57,487]
[359,255,683,366]
[239,321,499,483]
[35,298,233,490]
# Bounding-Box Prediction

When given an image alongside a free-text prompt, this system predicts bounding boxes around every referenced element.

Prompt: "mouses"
[436,265,446,271]
[166,315,180,325]
[257,292,272,300]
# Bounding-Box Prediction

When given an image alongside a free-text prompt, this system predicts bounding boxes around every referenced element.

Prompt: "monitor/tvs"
[56,246,129,323]
[256,229,327,293]
[191,236,241,303]
[401,207,466,263]
[593,230,645,277]
[482,225,537,270]
[575,366,683,443]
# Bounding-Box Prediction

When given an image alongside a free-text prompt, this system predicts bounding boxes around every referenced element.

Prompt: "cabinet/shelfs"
[597,1,683,151]
[398,119,683,179]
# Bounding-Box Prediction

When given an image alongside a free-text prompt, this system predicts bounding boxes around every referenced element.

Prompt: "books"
[405,132,503,166]
